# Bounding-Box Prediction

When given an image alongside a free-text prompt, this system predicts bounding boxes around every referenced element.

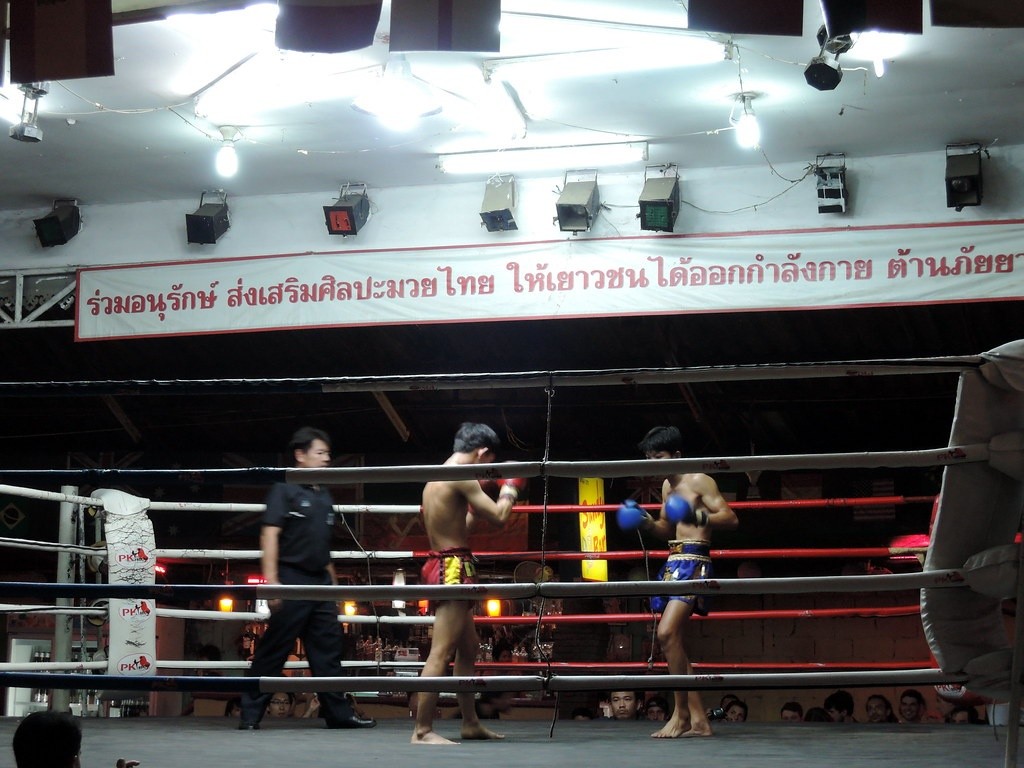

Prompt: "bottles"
[109,697,150,718]
[30,650,99,705]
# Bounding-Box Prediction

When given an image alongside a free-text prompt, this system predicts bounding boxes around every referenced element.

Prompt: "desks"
[190,692,559,718]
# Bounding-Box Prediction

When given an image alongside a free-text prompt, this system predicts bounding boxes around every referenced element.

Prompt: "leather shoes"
[328,716,377,729]
[238,724,260,730]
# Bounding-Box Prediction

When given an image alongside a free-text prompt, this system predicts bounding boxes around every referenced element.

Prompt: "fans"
[513,560,560,607]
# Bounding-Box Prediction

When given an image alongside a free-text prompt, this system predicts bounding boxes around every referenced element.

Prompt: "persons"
[181,641,370,718]
[409,641,524,720]
[780,689,988,723]
[238,426,377,730]
[617,425,739,739]
[12,711,141,768]
[410,422,527,746]
[571,690,748,722]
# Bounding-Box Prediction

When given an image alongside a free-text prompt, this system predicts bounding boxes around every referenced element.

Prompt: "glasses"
[270,699,290,705]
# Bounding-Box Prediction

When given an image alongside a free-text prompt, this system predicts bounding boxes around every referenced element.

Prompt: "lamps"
[733,92,762,150]
[222,560,236,612]
[215,124,238,178]
[391,567,407,610]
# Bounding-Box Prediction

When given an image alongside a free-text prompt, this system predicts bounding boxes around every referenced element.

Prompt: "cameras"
[708,708,728,721]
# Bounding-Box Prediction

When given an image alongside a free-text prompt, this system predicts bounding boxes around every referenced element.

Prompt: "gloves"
[617,499,653,530]
[665,494,708,528]
[496,461,526,498]
